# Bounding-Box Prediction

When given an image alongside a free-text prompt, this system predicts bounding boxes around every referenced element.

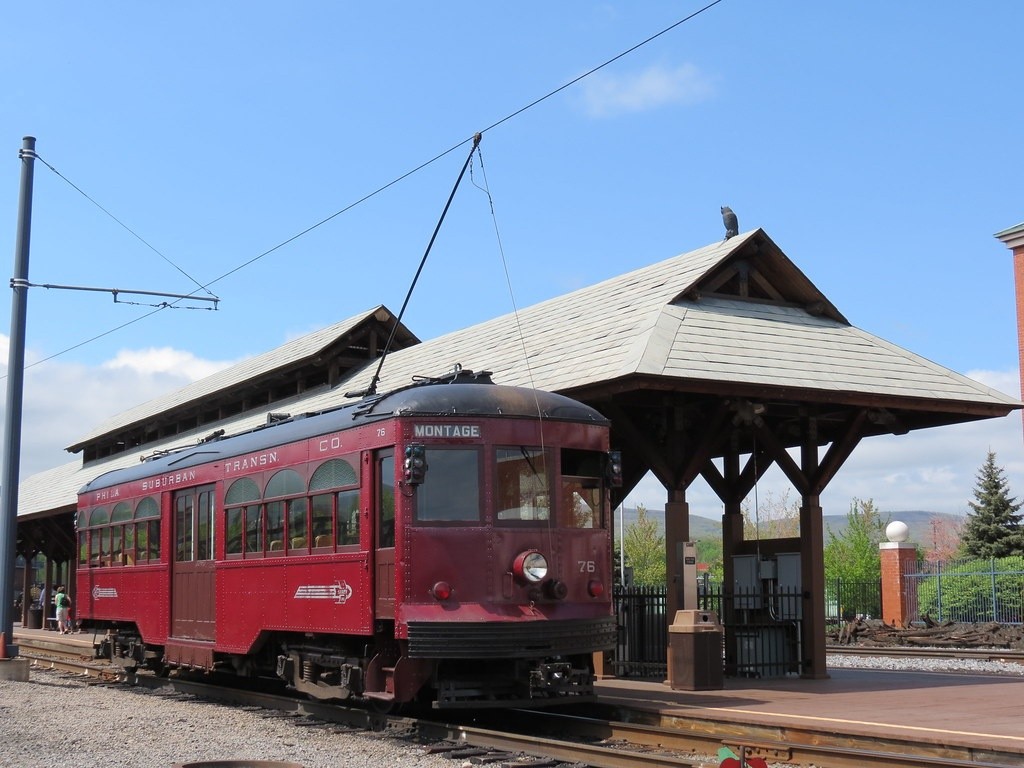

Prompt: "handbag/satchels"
[60,594,69,607]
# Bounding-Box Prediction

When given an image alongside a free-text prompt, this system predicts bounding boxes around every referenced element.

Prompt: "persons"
[39,587,54,630]
[63,607,73,633]
[54,586,71,635]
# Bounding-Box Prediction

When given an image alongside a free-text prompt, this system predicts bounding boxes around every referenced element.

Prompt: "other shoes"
[78,630,81,634]
[60,633,63,635]
[71,631,73,634]
[64,629,68,634]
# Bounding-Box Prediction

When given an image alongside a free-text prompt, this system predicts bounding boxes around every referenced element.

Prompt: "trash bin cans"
[27,608,43,629]
[668,607,726,691]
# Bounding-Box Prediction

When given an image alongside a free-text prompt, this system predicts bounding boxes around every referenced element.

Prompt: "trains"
[75,361,617,715]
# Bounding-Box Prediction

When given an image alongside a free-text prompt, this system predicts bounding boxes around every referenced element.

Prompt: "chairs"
[271,534,332,551]
[97,551,155,566]
